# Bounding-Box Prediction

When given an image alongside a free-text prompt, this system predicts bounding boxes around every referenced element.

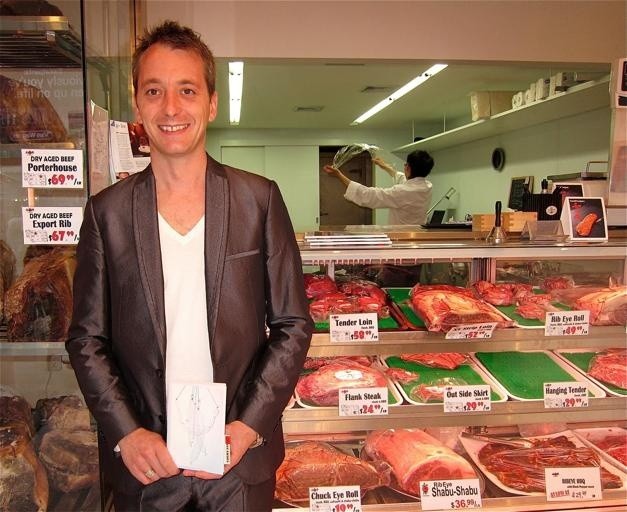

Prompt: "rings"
[144,469,154,479]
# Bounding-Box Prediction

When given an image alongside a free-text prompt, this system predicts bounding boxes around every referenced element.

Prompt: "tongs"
[462,431,541,448]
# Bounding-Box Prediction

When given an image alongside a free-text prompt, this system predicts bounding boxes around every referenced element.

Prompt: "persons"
[323,150,434,287]
[65,20,315,512]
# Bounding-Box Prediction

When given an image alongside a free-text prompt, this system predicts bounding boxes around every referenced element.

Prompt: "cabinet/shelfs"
[2,1,627,509]
